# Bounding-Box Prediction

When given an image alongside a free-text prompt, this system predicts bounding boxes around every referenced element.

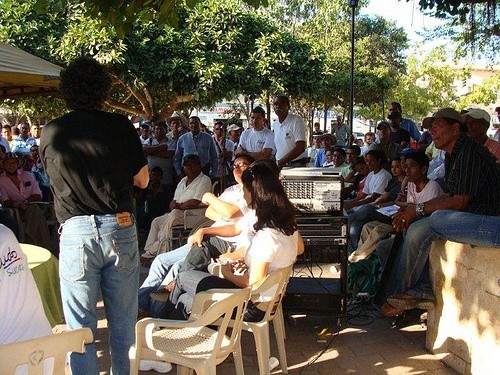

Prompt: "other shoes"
[140,254,155,262]
[380,293,415,317]
[348,259,372,271]
[388,293,437,310]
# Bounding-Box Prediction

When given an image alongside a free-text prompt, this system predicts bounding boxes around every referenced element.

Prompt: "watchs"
[415,202,427,218]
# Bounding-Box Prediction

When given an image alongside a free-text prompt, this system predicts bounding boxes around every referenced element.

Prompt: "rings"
[402,219,405,222]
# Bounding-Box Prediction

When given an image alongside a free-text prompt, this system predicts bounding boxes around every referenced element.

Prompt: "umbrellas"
[0,40,65,94]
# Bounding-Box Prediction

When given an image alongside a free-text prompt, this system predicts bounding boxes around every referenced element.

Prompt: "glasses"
[233,161,250,169]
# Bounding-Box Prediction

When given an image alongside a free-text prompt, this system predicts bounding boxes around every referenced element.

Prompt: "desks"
[18,243,64,327]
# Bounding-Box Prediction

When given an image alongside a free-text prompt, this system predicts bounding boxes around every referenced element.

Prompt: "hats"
[387,111,399,119]
[141,120,152,127]
[376,122,389,130]
[463,109,490,124]
[227,124,240,132]
[165,111,187,128]
[423,107,460,129]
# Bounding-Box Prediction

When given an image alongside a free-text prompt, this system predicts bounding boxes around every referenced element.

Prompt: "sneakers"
[138,358,172,373]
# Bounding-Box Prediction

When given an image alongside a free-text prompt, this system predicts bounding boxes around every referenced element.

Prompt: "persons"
[38,56,150,374]
[0,95,500,375]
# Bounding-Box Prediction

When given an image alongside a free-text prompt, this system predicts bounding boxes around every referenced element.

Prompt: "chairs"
[129,287,251,375]
[202,266,291,375]
[0,328,94,375]
